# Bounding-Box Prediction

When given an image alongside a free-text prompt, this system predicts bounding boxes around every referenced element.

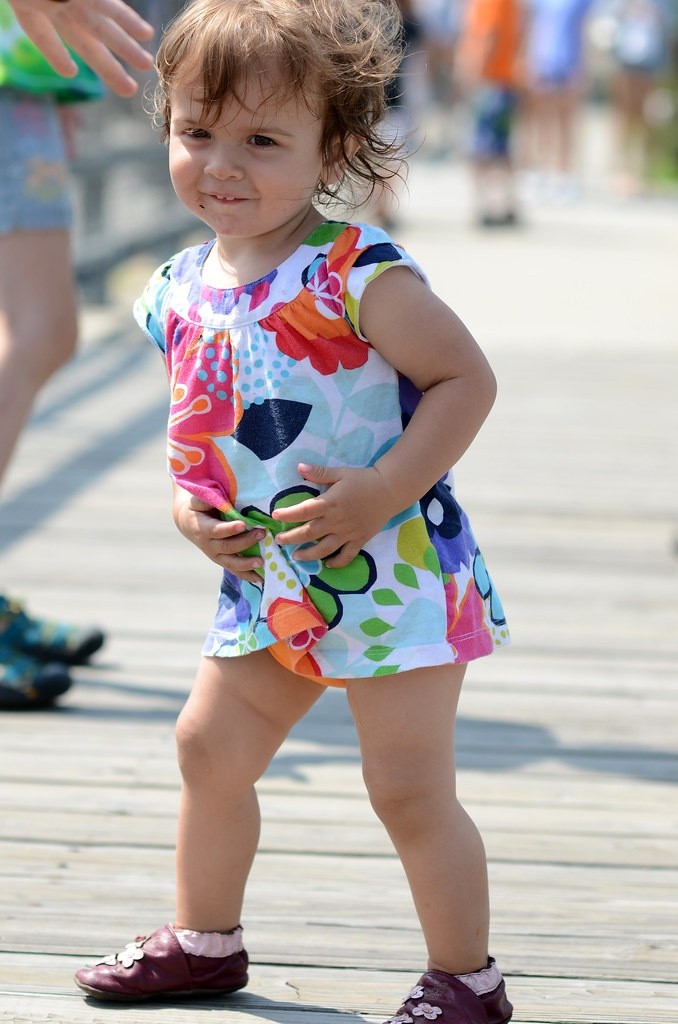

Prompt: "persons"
[0,1,156,712]
[353,1,665,234]
[74,1,516,1024]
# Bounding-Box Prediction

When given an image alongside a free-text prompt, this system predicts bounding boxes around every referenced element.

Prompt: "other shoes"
[0,642,72,709]
[74,924,249,1000]
[381,956,513,1024]
[480,210,516,227]
[0,596,103,661]
